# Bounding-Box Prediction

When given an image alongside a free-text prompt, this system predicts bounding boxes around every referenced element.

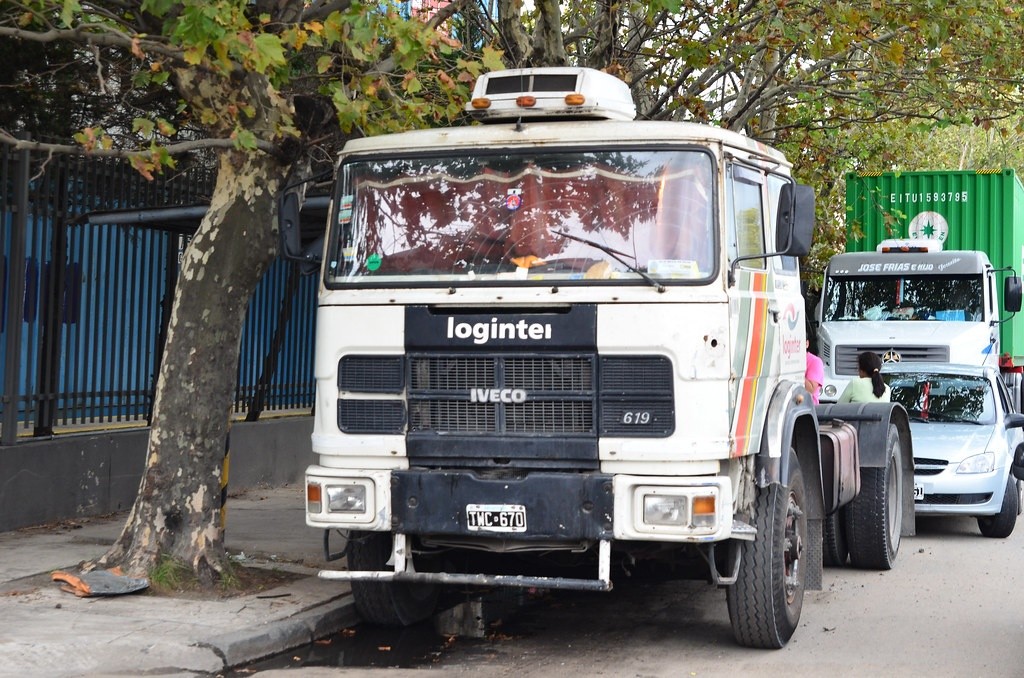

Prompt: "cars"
[870,361,1024,538]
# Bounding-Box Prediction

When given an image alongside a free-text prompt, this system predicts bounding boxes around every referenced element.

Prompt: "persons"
[805,332,824,405]
[837,352,891,404]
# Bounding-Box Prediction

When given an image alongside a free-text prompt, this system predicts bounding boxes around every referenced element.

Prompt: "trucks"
[275,68,916,650]
[807,167,1022,416]
